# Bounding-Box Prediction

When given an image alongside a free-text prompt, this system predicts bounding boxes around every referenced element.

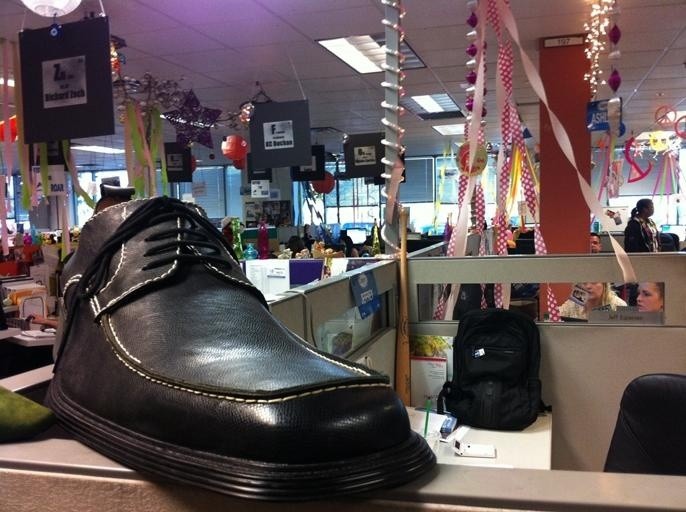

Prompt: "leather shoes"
[44,184,436,502]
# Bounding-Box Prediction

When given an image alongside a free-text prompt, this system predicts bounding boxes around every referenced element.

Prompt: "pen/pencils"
[41,329,56,333]
[424,396,431,437]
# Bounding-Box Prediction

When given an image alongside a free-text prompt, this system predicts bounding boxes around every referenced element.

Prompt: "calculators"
[452,439,497,458]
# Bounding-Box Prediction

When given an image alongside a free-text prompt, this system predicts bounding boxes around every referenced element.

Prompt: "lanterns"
[313,172,335,194]
[221,135,248,169]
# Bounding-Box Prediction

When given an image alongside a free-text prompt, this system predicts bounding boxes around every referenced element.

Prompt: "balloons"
[456,140,488,176]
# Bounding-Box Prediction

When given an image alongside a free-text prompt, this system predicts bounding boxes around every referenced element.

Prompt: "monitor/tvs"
[346,228,367,246]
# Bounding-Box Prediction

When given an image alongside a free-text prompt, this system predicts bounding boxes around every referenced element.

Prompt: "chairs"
[604,373,686,476]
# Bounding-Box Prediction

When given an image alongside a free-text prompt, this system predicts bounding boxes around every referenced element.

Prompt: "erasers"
[440,418,457,433]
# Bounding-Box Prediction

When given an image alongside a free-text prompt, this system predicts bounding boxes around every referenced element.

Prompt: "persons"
[559,199,664,321]
[286,235,312,258]
[344,236,359,257]
[32,315,58,329]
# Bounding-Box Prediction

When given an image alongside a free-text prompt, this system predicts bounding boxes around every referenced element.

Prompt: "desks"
[407,400,554,470]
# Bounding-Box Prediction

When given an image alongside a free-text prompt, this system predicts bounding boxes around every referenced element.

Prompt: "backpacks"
[437,307,552,431]
